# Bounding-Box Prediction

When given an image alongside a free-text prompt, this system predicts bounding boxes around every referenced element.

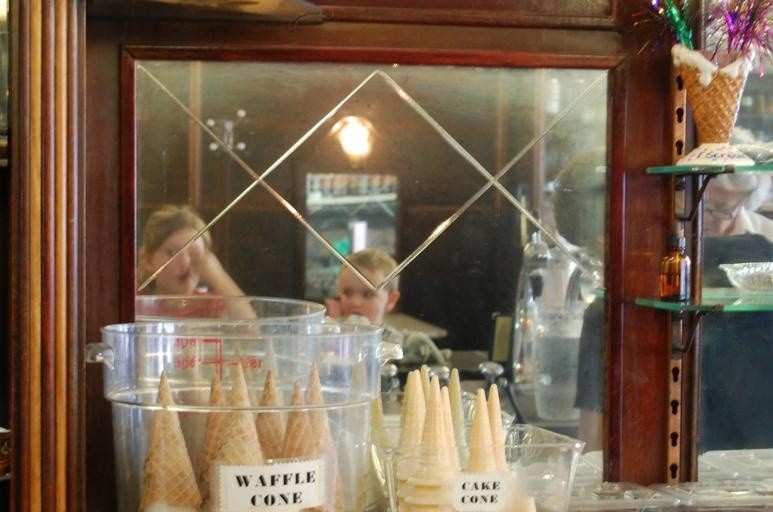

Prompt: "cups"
[530,294,590,422]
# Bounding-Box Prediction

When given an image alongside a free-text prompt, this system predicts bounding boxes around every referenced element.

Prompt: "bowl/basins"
[718,261,772,294]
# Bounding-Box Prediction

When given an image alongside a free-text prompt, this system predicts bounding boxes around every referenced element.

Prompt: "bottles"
[657,235,693,302]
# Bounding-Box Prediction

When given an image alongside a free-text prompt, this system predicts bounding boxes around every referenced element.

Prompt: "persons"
[137,205,263,349]
[340,248,451,394]
[677,126,772,244]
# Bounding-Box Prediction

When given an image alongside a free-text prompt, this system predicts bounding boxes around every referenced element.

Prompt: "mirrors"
[118,40,631,487]
